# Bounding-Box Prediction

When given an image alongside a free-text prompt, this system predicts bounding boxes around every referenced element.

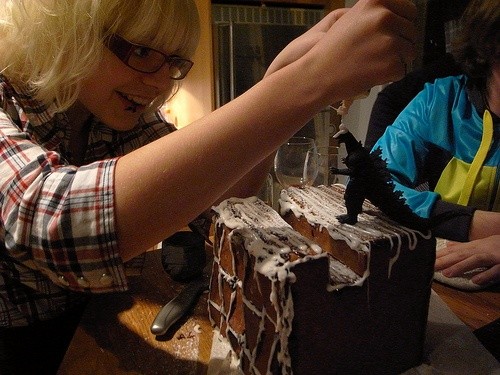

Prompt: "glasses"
[99,27,194,80]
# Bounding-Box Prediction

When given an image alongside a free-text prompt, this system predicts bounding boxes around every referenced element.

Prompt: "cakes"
[209,183,436,375]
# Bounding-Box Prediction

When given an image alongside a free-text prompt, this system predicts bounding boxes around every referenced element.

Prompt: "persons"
[0,0,420,375]
[362,0,457,195]
[370,0,500,286]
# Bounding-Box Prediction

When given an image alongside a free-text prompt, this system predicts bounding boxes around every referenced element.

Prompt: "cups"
[303,145,350,189]
[161,231,207,280]
[274,136,319,190]
[256,173,274,208]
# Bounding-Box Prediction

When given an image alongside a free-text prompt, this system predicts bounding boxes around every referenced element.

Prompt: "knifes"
[151,259,213,335]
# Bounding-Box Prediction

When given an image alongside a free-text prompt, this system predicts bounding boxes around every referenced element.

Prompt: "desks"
[56,224,500,375]
